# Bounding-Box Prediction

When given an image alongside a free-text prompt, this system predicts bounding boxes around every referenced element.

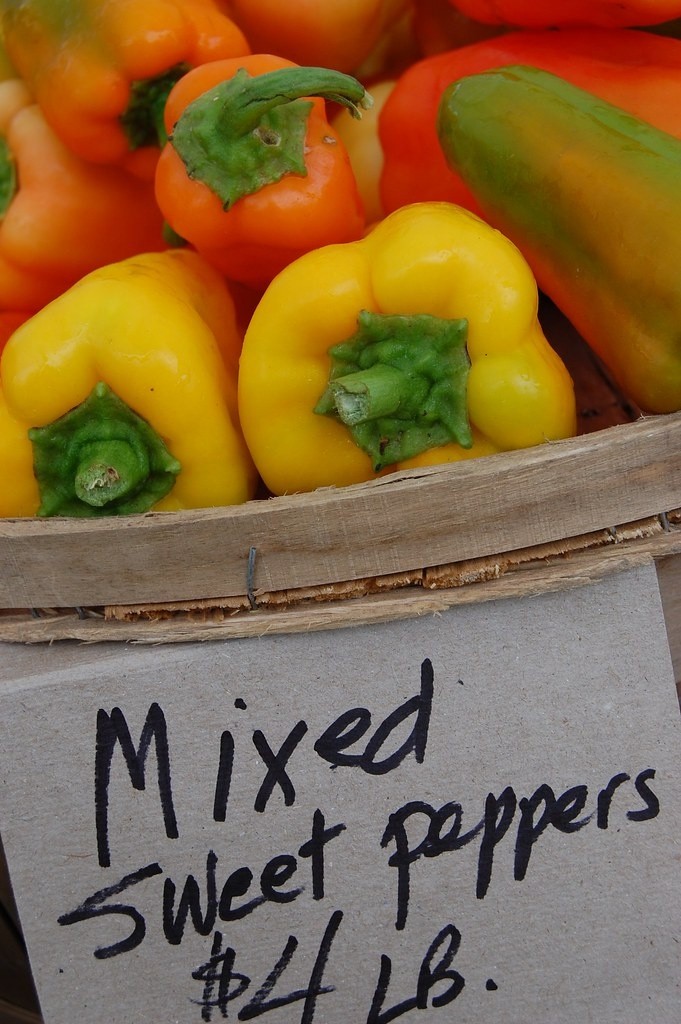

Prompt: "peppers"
[0,0,681,519]
